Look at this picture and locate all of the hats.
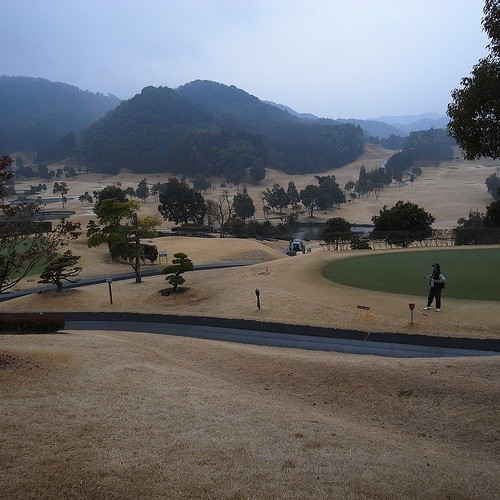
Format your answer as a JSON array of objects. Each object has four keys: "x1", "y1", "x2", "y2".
[{"x1": 432, "y1": 263, "x2": 440, "y2": 268}]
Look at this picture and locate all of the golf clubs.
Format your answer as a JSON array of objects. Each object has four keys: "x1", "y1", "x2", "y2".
[{"x1": 424, "y1": 275, "x2": 435, "y2": 281}]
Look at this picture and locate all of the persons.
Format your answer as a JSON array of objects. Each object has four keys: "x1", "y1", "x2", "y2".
[
  {"x1": 300, "y1": 242, "x2": 312, "y2": 253},
  {"x1": 288, "y1": 242, "x2": 292, "y2": 251},
  {"x1": 423, "y1": 263, "x2": 446, "y2": 312}
]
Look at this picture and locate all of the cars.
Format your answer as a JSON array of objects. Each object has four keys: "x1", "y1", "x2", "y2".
[{"x1": 293, "y1": 239, "x2": 301, "y2": 245}]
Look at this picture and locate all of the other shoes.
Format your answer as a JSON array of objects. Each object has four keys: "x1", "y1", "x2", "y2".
[
  {"x1": 423, "y1": 306, "x2": 432, "y2": 310},
  {"x1": 435, "y1": 308, "x2": 441, "y2": 312}
]
